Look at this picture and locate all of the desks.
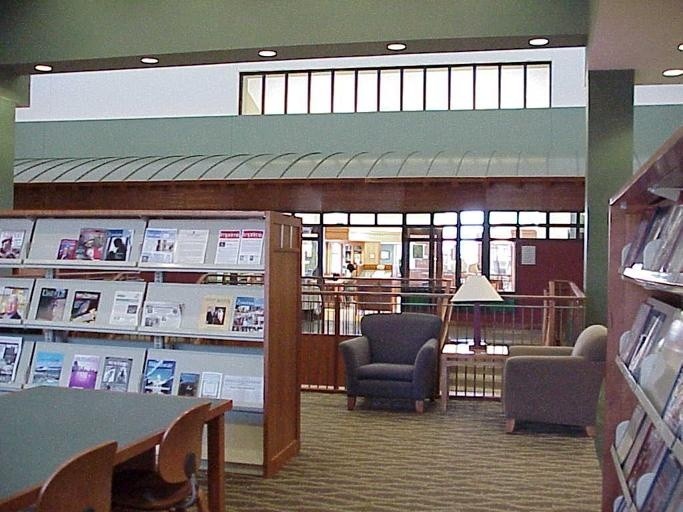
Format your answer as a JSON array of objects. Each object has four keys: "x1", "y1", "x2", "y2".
[
  {"x1": 440, "y1": 344, "x2": 510, "y2": 416},
  {"x1": 0, "y1": 385, "x2": 232, "y2": 511}
]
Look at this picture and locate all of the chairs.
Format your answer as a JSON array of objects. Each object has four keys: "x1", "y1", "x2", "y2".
[
  {"x1": 501, "y1": 324, "x2": 608, "y2": 436},
  {"x1": 111, "y1": 401, "x2": 212, "y2": 510},
  {"x1": 338, "y1": 312, "x2": 443, "y2": 414},
  {"x1": 36, "y1": 441, "x2": 118, "y2": 511}
]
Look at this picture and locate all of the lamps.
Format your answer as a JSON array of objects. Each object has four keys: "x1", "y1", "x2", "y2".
[{"x1": 449, "y1": 275, "x2": 505, "y2": 352}]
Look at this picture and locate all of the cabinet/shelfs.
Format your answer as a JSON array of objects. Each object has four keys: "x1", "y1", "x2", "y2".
[
  {"x1": 601, "y1": 125, "x2": 682, "y2": 512},
  {"x1": 1, "y1": 209, "x2": 302, "y2": 479},
  {"x1": 324, "y1": 241, "x2": 366, "y2": 277}
]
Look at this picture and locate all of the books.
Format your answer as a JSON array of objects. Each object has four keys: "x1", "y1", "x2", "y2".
[
  {"x1": 2, "y1": 226, "x2": 134, "y2": 262},
  {"x1": 615, "y1": 190, "x2": 683, "y2": 511},
  {"x1": 0, "y1": 336, "x2": 224, "y2": 398},
  {"x1": 0, "y1": 285, "x2": 264, "y2": 333}
]
